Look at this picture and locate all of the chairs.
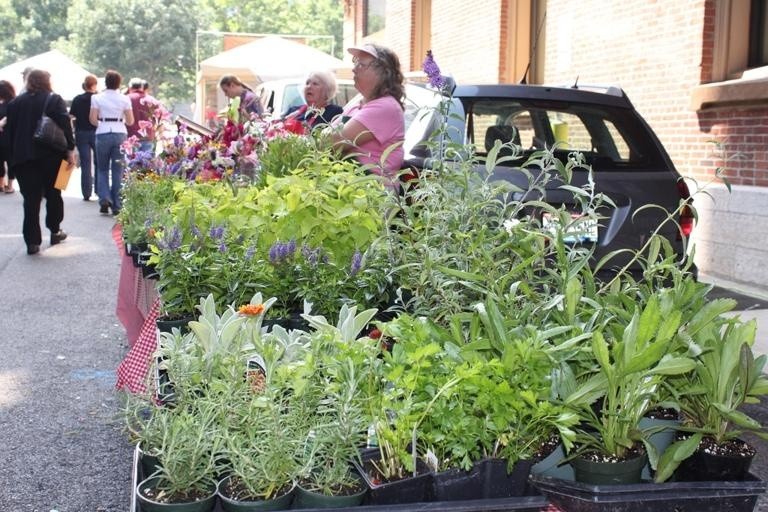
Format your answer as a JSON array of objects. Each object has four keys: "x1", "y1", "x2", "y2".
[{"x1": 484, "y1": 123, "x2": 523, "y2": 159}]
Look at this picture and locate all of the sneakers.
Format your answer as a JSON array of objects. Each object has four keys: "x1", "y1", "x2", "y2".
[
  {"x1": 26, "y1": 244, "x2": 41, "y2": 255},
  {"x1": 51, "y1": 229, "x2": 67, "y2": 245},
  {"x1": 100, "y1": 202, "x2": 110, "y2": 214}
]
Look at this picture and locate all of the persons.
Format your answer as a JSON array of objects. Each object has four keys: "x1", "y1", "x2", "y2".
[
  {"x1": 18, "y1": 68, "x2": 35, "y2": 94},
  {"x1": 139, "y1": 79, "x2": 163, "y2": 158},
  {"x1": 88, "y1": 70, "x2": 135, "y2": 214},
  {"x1": 317, "y1": 44, "x2": 404, "y2": 197},
  {"x1": 125, "y1": 78, "x2": 169, "y2": 165},
  {"x1": 68, "y1": 76, "x2": 97, "y2": 203},
  {"x1": 0, "y1": 80, "x2": 16, "y2": 194},
  {"x1": 221, "y1": 76, "x2": 263, "y2": 125},
  {"x1": 282, "y1": 70, "x2": 343, "y2": 134},
  {"x1": 4, "y1": 70, "x2": 75, "y2": 255}
]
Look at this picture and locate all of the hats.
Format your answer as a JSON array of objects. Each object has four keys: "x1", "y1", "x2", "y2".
[{"x1": 348, "y1": 43, "x2": 378, "y2": 59}]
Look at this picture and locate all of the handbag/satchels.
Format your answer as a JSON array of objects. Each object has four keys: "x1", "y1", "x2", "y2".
[{"x1": 33, "y1": 115, "x2": 68, "y2": 153}]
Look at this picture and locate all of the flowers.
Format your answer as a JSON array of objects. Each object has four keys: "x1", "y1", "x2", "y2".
[{"x1": 144, "y1": 202, "x2": 366, "y2": 313}]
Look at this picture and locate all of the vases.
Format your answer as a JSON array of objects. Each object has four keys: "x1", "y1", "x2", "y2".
[
  {"x1": 132, "y1": 183, "x2": 165, "y2": 267},
  {"x1": 124, "y1": 178, "x2": 146, "y2": 257},
  {"x1": 156, "y1": 313, "x2": 316, "y2": 335}
]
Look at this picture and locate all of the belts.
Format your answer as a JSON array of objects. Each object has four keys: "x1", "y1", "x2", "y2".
[{"x1": 99, "y1": 118, "x2": 122, "y2": 121}]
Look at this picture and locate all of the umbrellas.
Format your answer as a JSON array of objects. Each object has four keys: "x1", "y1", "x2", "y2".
[
  {"x1": 200, "y1": 32, "x2": 354, "y2": 73},
  {"x1": 1, "y1": 49, "x2": 110, "y2": 102}
]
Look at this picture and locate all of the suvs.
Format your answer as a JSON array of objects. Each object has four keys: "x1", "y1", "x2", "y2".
[{"x1": 340, "y1": 81, "x2": 703, "y2": 310}]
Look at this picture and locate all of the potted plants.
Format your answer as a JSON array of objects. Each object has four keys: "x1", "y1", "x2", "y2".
[{"x1": 141, "y1": 177, "x2": 175, "y2": 278}]
